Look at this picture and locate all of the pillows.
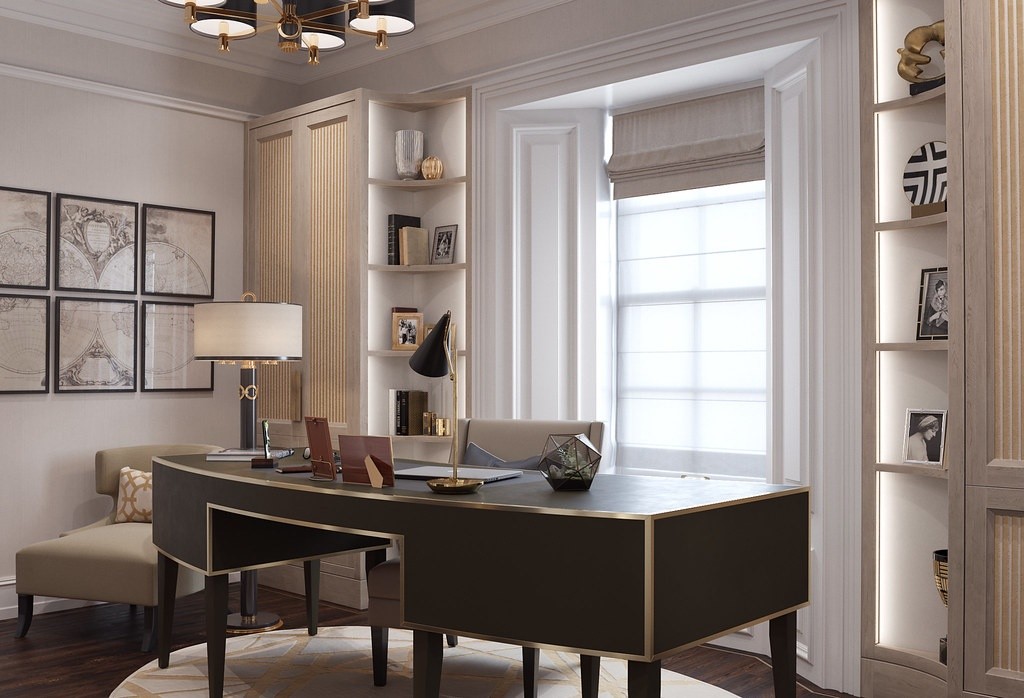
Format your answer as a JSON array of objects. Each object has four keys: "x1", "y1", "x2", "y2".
[{"x1": 114, "y1": 466, "x2": 153, "y2": 523}]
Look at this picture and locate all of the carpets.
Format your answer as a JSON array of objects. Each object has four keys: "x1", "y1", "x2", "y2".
[{"x1": 110, "y1": 626, "x2": 742, "y2": 698}]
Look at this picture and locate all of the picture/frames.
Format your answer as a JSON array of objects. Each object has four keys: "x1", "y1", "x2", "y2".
[
  {"x1": 430, "y1": 224, "x2": 458, "y2": 264},
  {"x1": 337, "y1": 435, "x2": 396, "y2": 488},
  {"x1": 0, "y1": 294, "x2": 51, "y2": 394},
  {"x1": 304, "y1": 416, "x2": 337, "y2": 480},
  {"x1": 141, "y1": 203, "x2": 216, "y2": 300},
  {"x1": 140, "y1": 301, "x2": 214, "y2": 392},
  {"x1": 916, "y1": 267, "x2": 948, "y2": 340},
  {"x1": 423, "y1": 323, "x2": 450, "y2": 350},
  {"x1": 54, "y1": 193, "x2": 139, "y2": 295},
  {"x1": 54, "y1": 296, "x2": 138, "y2": 393},
  {"x1": 392, "y1": 312, "x2": 423, "y2": 351},
  {"x1": 0, "y1": 186, "x2": 51, "y2": 290},
  {"x1": 901, "y1": 408, "x2": 948, "y2": 466}
]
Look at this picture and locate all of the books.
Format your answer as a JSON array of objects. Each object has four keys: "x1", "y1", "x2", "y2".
[
  {"x1": 206, "y1": 449, "x2": 294, "y2": 462},
  {"x1": 389, "y1": 388, "x2": 450, "y2": 435},
  {"x1": 387, "y1": 214, "x2": 430, "y2": 265},
  {"x1": 276, "y1": 465, "x2": 312, "y2": 473}
]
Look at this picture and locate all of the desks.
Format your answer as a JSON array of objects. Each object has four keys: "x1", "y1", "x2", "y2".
[{"x1": 151, "y1": 447, "x2": 811, "y2": 698}]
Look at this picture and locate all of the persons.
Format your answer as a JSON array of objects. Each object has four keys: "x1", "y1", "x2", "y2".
[
  {"x1": 399, "y1": 321, "x2": 416, "y2": 344},
  {"x1": 439, "y1": 233, "x2": 448, "y2": 256},
  {"x1": 926, "y1": 280, "x2": 948, "y2": 329},
  {"x1": 908, "y1": 415, "x2": 939, "y2": 462}
]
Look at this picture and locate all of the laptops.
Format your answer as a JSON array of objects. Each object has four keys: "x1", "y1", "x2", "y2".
[{"x1": 393, "y1": 465, "x2": 523, "y2": 482}]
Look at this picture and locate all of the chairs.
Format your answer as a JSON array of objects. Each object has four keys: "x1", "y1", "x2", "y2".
[
  {"x1": 13, "y1": 444, "x2": 227, "y2": 653},
  {"x1": 366, "y1": 418, "x2": 605, "y2": 698}
]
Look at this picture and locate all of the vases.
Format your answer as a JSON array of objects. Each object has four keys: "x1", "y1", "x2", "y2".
[{"x1": 395, "y1": 129, "x2": 424, "y2": 181}]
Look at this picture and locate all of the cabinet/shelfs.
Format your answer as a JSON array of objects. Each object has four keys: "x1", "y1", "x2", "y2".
[
  {"x1": 244, "y1": 85, "x2": 471, "y2": 454},
  {"x1": 859, "y1": 0, "x2": 1024, "y2": 698}
]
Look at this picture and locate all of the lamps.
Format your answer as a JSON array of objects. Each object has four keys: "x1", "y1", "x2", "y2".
[
  {"x1": 158, "y1": 0, "x2": 417, "y2": 66},
  {"x1": 194, "y1": 291, "x2": 303, "y2": 634},
  {"x1": 408, "y1": 309, "x2": 484, "y2": 494}
]
